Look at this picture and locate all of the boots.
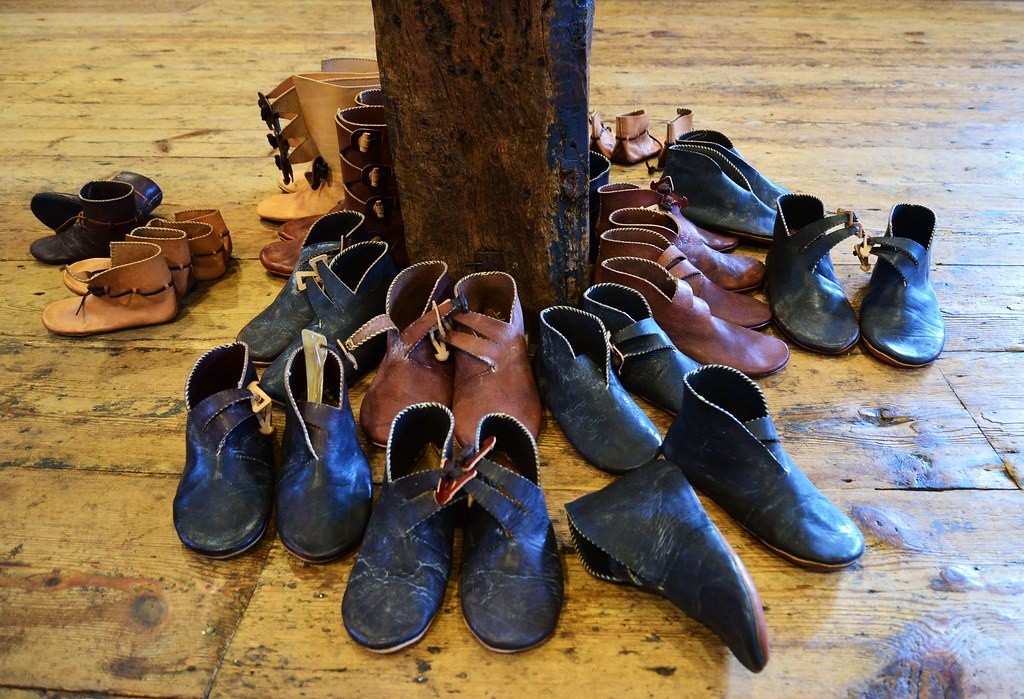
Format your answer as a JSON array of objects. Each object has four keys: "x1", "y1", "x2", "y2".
[{"x1": 31, "y1": 170, "x2": 232, "y2": 333}]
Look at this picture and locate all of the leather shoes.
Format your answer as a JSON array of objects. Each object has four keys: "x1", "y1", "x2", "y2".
[{"x1": 173, "y1": 58, "x2": 945, "y2": 672}]
